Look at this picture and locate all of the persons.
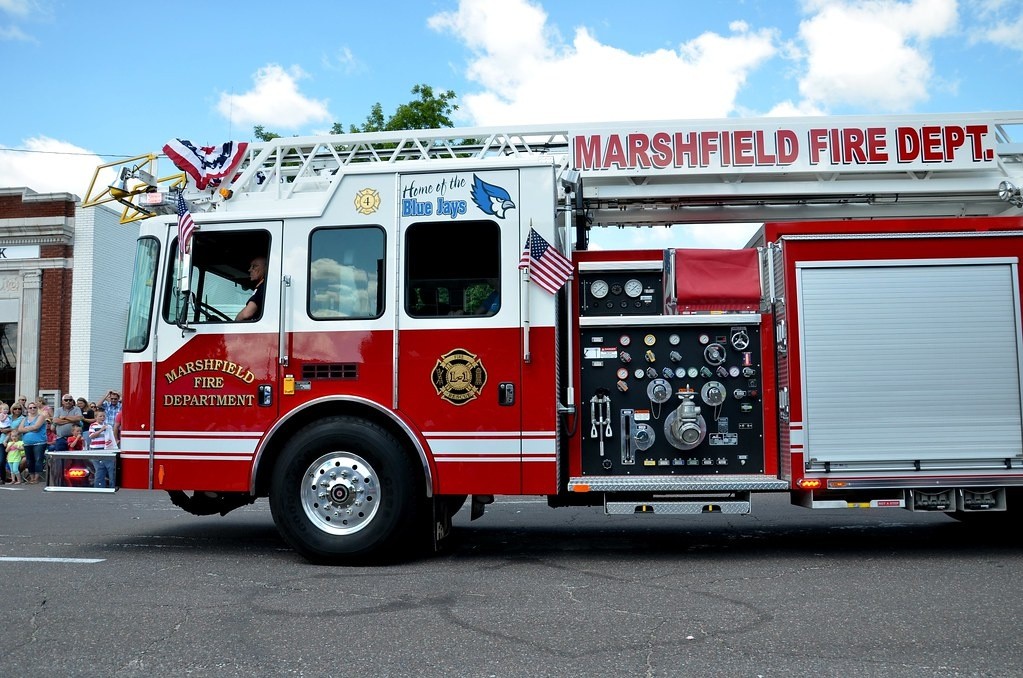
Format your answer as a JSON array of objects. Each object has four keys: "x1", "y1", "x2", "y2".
[
  {"x1": 89, "y1": 408, "x2": 120, "y2": 492},
  {"x1": 35, "y1": 397, "x2": 57, "y2": 452},
  {"x1": 68, "y1": 424, "x2": 84, "y2": 451},
  {"x1": 5, "y1": 430, "x2": 24, "y2": 484},
  {"x1": 77, "y1": 398, "x2": 96, "y2": 444},
  {"x1": 113, "y1": 412, "x2": 121, "y2": 439},
  {"x1": 0, "y1": 401, "x2": 24, "y2": 485},
  {"x1": 98, "y1": 390, "x2": 122, "y2": 426},
  {"x1": 52, "y1": 394, "x2": 83, "y2": 450},
  {"x1": 478, "y1": 279, "x2": 500, "y2": 315},
  {"x1": 17, "y1": 395, "x2": 28, "y2": 417},
  {"x1": 18, "y1": 402, "x2": 47, "y2": 484},
  {"x1": 235, "y1": 256, "x2": 266, "y2": 321}
]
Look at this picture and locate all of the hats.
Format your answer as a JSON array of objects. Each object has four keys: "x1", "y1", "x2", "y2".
[
  {"x1": 110, "y1": 390, "x2": 121, "y2": 399},
  {"x1": 45, "y1": 418, "x2": 51, "y2": 423},
  {"x1": 72, "y1": 422, "x2": 82, "y2": 427}
]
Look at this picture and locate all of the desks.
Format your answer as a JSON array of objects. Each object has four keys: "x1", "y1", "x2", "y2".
[{"x1": 44, "y1": 450, "x2": 119, "y2": 493}]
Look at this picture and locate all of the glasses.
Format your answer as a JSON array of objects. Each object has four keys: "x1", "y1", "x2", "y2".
[
  {"x1": 20, "y1": 399, "x2": 26, "y2": 401},
  {"x1": 91, "y1": 406, "x2": 96, "y2": 408},
  {"x1": 13, "y1": 408, "x2": 21, "y2": 410},
  {"x1": 111, "y1": 397, "x2": 118, "y2": 400},
  {"x1": 48, "y1": 423, "x2": 50, "y2": 424},
  {"x1": 29, "y1": 407, "x2": 36, "y2": 409},
  {"x1": 64, "y1": 399, "x2": 73, "y2": 402},
  {"x1": 249, "y1": 264, "x2": 265, "y2": 269}
]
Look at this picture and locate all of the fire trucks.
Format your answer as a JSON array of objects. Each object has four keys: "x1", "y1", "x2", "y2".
[{"x1": 82, "y1": 111, "x2": 1023, "y2": 566}]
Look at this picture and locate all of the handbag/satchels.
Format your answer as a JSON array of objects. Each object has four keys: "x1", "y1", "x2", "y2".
[{"x1": 104, "y1": 424, "x2": 119, "y2": 450}]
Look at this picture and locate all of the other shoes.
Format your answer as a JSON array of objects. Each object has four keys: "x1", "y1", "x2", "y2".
[
  {"x1": 28, "y1": 479, "x2": 39, "y2": 484},
  {"x1": 9, "y1": 480, "x2": 21, "y2": 485},
  {"x1": 3, "y1": 441, "x2": 8, "y2": 447},
  {"x1": 2, "y1": 480, "x2": 5, "y2": 484}
]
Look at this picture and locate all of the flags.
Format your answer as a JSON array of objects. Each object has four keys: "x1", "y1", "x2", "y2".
[
  {"x1": 177, "y1": 191, "x2": 195, "y2": 253},
  {"x1": 518, "y1": 227, "x2": 574, "y2": 295}
]
